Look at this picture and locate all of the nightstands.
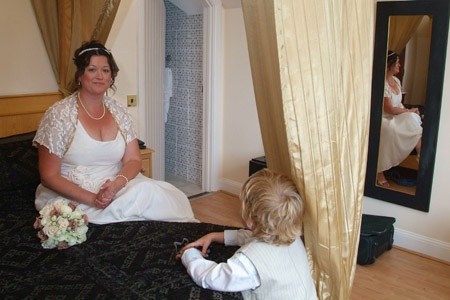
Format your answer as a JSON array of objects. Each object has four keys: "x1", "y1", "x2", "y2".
[{"x1": 135, "y1": 147, "x2": 155, "y2": 178}]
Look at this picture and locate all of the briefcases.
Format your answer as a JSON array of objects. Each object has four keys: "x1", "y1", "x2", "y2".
[{"x1": 357, "y1": 213, "x2": 396, "y2": 265}]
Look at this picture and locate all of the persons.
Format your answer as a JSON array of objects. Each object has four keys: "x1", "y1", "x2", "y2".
[
  {"x1": 376, "y1": 51, "x2": 423, "y2": 188},
  {"x1": 33, "y1": 40, "x2": 202, "y2": 224},
  {"x1": 176, "y1": 167, "x2": 319, "y2": 300}
]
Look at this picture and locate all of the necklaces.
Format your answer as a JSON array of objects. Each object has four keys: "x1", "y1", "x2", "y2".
[
  {"x1": 77, "y1": 90, "x2": 105, "y2": 120},
  {"x1": 386, "y1": 76, "x2": 395, "y2": 86}
]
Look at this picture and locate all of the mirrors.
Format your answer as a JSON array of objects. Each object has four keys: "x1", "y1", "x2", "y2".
[{"x1": 363, "y1": 0, "x2": 450, "y2": 212}]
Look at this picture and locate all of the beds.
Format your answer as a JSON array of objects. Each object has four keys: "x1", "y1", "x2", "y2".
[{"x1": 0, "y1": 92, "x2": 252, "y2": 300}]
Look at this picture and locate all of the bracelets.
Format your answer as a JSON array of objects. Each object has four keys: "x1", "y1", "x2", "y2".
[
  {"x1": 116, "y1": 175, "x2": 128, "y2": 187},
  {"x1": 407, "y1": 108, "x2": 409, "y2": 112}
]
